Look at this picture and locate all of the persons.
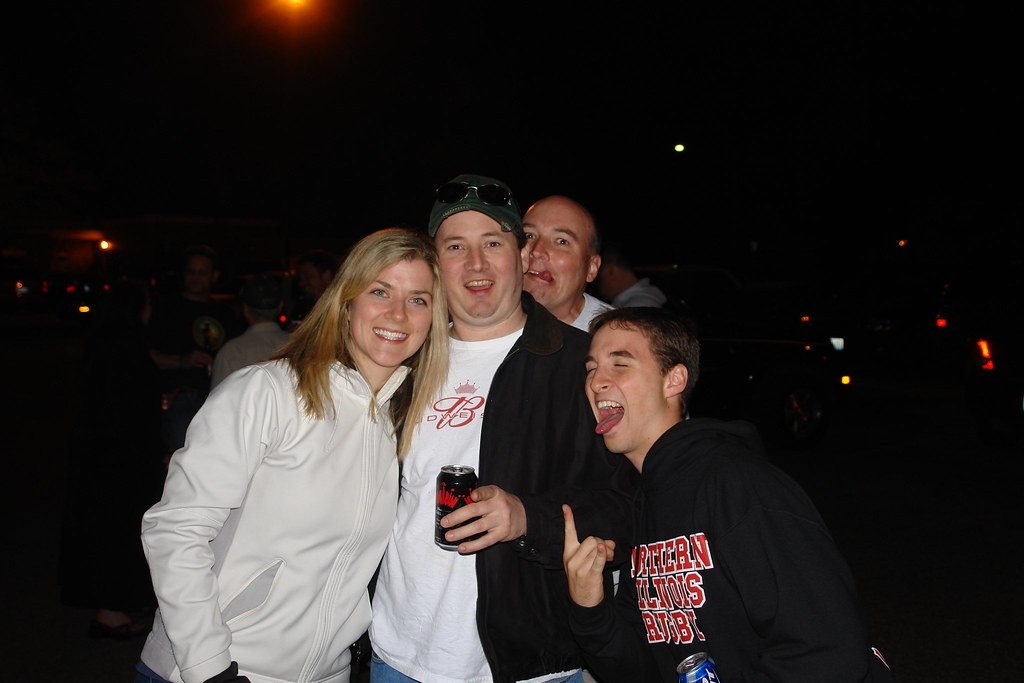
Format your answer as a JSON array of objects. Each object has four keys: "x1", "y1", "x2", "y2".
[
  {"x1": 130, "y1": 230, "x2": 449, "y2": 683},
  {"x1": 366, "y1": 177, "x2": 608, "y2": 683},
  {"x1": 563, "y1": 306, "x2": 903, "y2": 683},
  {"x1": 59, "y1": 242, "x2": 670, "y2": 638}
]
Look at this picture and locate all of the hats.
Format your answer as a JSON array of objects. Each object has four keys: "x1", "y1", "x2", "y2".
[{"x1": 429, "y1": 175, "x2": 523, "y2": 238}]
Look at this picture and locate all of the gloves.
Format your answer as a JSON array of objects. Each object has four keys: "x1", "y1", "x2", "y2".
[{"x1": 203, "y1": 662, "x2": 251, "y2": 683}]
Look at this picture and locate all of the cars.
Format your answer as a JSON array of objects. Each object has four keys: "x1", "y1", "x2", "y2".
[
  {"x1": 1, "y1": 225, "x2": 181, "y2": 331},
  {"x1": 650, "y1": 258, "x2": 861, "y2": 448}
]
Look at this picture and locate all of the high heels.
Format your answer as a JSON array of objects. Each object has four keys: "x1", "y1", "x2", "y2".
[{"x1": 88, "y1": 620, "x2": 144, "y2": 640}]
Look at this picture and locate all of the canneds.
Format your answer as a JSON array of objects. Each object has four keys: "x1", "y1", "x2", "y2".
[
  {"x1": 676, "y1": 652, "x2": 723, "y2": 683},
  {"x1": 434, "y1": 465, "x2": 479, "y2": 550}
]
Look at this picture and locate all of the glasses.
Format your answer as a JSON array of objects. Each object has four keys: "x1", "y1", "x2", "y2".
[{"x1": 436, "y1": 181, "x2": 513, "y2": 207}]
